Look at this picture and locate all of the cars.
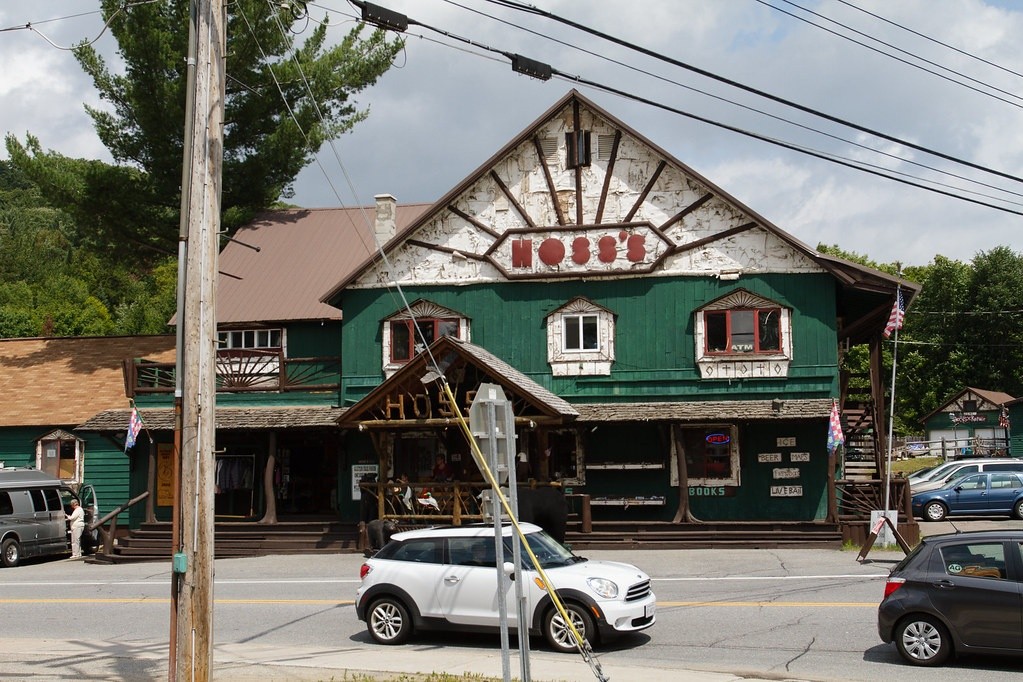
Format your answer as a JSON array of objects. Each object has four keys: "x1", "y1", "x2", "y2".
[
  {"x1": 911, "y1": 470, "x2": 1023, "y2": 519},
  {"x1": 904, "y1": 457, "x2": 1023, "y2": 486}
]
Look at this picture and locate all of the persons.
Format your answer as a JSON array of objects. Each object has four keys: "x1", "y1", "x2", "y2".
[
  {"x1": 64, "y1": 499, "x2": 84, "y2": 558},
  {"x1": 396, "y1": 472, "x2": 408, "y2": 484},
  {"x1": 433, "y1": 454, "x2": 454, "y2": 482}
]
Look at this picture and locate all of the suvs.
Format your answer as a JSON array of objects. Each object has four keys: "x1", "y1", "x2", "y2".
[
  {"x1": 356, "y1": 523, "x2": 659, "y2": 654},
  {"x1": 878, "y1": 532, "x2": 1023, "y2": 668}
]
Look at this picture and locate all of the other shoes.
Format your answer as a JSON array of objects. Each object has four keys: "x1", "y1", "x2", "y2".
[{"x1": 70, "y1": 555, "x2": 82, "y2": 559}]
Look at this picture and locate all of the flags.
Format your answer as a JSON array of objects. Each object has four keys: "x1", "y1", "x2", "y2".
[
  {"x1": 124, "y1": 407, "x2": 142, "y2": 453},
  {"x1": 827, "y1": 402, "x2": 844, "y2": 455},
  {"x1": 882, "y1": 290, "x2": 904, "y2": 339}
]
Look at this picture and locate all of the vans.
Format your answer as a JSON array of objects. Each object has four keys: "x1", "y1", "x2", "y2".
[
  {"x1": 908, "y1": 461, "x2": 1023, "y2": 496},
  {"x1": 0, "y1": 470, "x2": 99, "y2": 567}
]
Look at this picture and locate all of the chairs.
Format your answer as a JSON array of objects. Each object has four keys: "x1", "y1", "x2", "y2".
[{"x1": 453, "y1": 549, "x2": 473, "y2": 564}]
